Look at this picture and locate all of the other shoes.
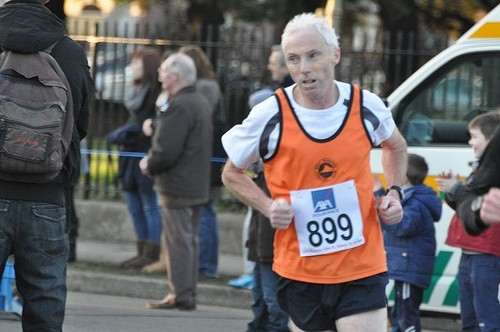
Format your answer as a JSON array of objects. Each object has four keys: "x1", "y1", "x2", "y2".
[
  {"x1": 147, "y1": 297, "x2": 191, "y2": 310},
  {"x1": 144, "y1": 259, "x2": 167, "y2": 273},
  {"x1": 198, "y1": 273, "x2": 215, "y2": 283}
]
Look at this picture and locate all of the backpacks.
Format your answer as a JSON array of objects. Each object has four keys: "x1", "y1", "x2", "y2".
[{"x1": 0, "y1": 34, "x2": 74, "y2": 184}]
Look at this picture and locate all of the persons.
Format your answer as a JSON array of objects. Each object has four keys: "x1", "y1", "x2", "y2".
[
  {"x1": 372, "y1": 153, "x2": 442, "y2": 332},
  {"x1": 106, "y1": 12, "x2": 409, "y2": 332},
  {"x1": 456, "y1": 126, "x2": 500, "y2": 236},
  {"x1": 0, "y1": 0, "x2": 97, "y2": 332},
  {"x1": 444, "y1": 110, "x2": 500, "y2": 332}
]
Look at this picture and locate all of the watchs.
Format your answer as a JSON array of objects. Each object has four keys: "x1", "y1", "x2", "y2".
[
  {"x1": 470, "y1": 195, "x2": 491, "y2": 231},
  {"x1": 384, "y1": 186, "x2": 405, "y2": 201}
]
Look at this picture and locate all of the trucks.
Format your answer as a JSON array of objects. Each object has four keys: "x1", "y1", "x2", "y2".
[{"x1": 369, "y1": 5, "x2": 500, "y2": 315}]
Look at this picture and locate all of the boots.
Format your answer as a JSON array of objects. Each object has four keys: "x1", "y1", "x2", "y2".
[{"x1": 121, "y1": 240, "x2": 160, "y2": 269}]
